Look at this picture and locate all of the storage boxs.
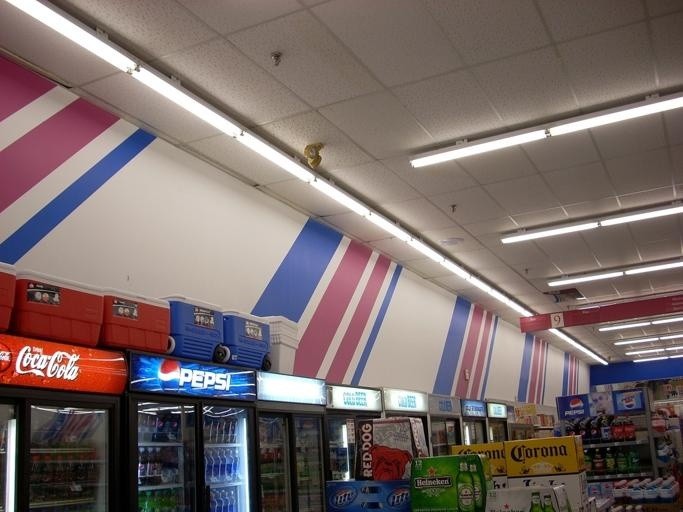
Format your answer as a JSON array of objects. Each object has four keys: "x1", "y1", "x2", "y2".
[{"x1": 323, "y1": 429, "x2": 595, "y2": 512}]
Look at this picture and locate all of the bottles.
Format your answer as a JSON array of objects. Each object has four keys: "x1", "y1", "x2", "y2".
[
  {"x1": 259, "y1": 441, "x2": 310, "y2": 512},
  {"x1": 29, "y1": 451, "x2": 97, "y2": 504},
  {"x1": 555, "y1": 414, "x2": 640, "y2": 476},
  {"x1": 454, "y1": 455, "x2": 484, "y2": 511},
  {"x1": 132, "y1": 407, "x2": 237, "y2": 511}
]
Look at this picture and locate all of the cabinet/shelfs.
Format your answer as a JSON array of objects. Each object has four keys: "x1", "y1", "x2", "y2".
[{"x1": 506, "y1": 388, "x2": 683, "y2": 510}]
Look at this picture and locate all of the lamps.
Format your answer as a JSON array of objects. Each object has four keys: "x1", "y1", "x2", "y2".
[
  {"x1": 132, "y1": 67, "x2": 241, "y2": 138},
  {"x1": 239, "y1": 133, "x2": 315, "y2": 182},
  {"x1": 408, "y1": 126, "x2": 547, "y2": 168},
  {"x1": 623, "y1": 260, "x2": 683, "y2": 276},
  {"x1": 310, "y1": 179, "x2": 369, "y2": 217},
  {"x1": 2, "y1": 0, "x2": 137, "y2": 74},
  {"x1": 546, "y1": 271, "x2": 624, "y2": 288},
  {"x1": 547, "y1": 317, "x2": 681, "y2": 363},
  {"x1": 549, "y1": 91, "x2": 683, "y2": 137},
  {"x1": 406, "y1": 238, "x2": 534, "y2": 321},
  {"x1": 368, "y1": 213, "x2": 412, "y2": 244},
  {"x1": 499, "y1": 219, "x2": 599, "y2": 245},
  {"x1": 599, "y1": 205, "x2": 683, "y2": 228}
]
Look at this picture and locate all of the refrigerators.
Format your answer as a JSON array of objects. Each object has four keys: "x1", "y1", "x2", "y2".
[
  {"x1": 0, "y1": 334, "x2": 128, "y2": 512},
  {"x1": 331, "y1": 381, "x2": 385, "y2": 510},
  {"x1": 462, "y1": 400, "x2": 488, "y2": 448},
  {"x1": 387, "y1": 386, "x2": 429, "y2": 450},
  {"x1": 129, "y1": 361, "x2": 256, "y2": 512},
  {"x1": 257, "y1": 377, "x2": 330, "y2": 508},
  {"x1": 427, "y1": 395, "x2": 461, "y2": 455},
  {"x1": 489, "y1": 401, "x2": 515, "y2": 441}
]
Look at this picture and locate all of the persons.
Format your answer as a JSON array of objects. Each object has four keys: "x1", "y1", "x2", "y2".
[{"x1": 590, "y1": 392, "x2": 612, "y2": 415}]
